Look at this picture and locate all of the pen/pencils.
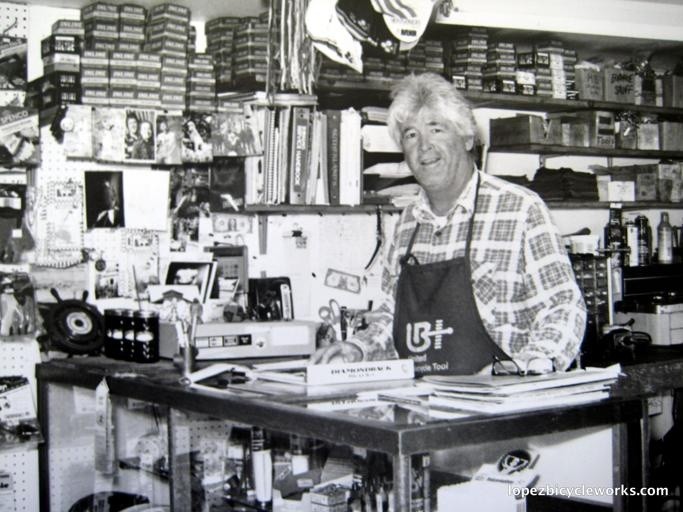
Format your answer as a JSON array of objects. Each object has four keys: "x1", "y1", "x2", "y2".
[{"x1": 339, "y1": 307, "x2": 349, "y2": 347}]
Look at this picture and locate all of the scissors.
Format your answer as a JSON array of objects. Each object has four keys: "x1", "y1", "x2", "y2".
[{"x1": 320, "y1": 300, "x2": 342, "y2": 342}]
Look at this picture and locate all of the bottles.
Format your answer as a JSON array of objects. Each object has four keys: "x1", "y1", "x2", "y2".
[
  {"x1": 344, "y1": 480, "x2": 388, "y2": 511},
  {"x1": 603, "y1": 204, "x2": 675, "y2": 268}
]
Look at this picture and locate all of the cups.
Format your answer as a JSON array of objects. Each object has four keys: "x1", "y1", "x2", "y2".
[{"x1": 102, "y1": 308, "x2": 160, "y2": 364}]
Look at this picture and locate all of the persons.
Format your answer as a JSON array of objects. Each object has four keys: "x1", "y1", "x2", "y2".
[
  {"x1": 126, "y1": 112, "x2": 154, "y2": 159},
  {"x1": 95, "y1": 176, "x2": 115, "y2": 225},
  {"x1": 157, "y1": 119, "x2": 175, "y2": 165},
  {"x1": 307, "y1": 71, "x2": 587, "y2": 376},
  {"x1": 0, "y1": 276, "x2": 34, "y2": 336}
]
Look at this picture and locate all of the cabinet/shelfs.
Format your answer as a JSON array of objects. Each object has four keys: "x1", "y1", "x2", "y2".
[
  {"x1": 36, "y1": 362, "x2": 644, "y2": 512},
  {"x1": 241, "y1": 22, "x2": 683, "y2": 214}
]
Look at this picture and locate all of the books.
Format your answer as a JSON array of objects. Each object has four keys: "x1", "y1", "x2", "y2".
[
  {"x1": 212, "y1": 90, "x2": 419, "y2": 207},
  {"x1": 380, "y1": 363, "x2": 620, "y2": 422}
]
